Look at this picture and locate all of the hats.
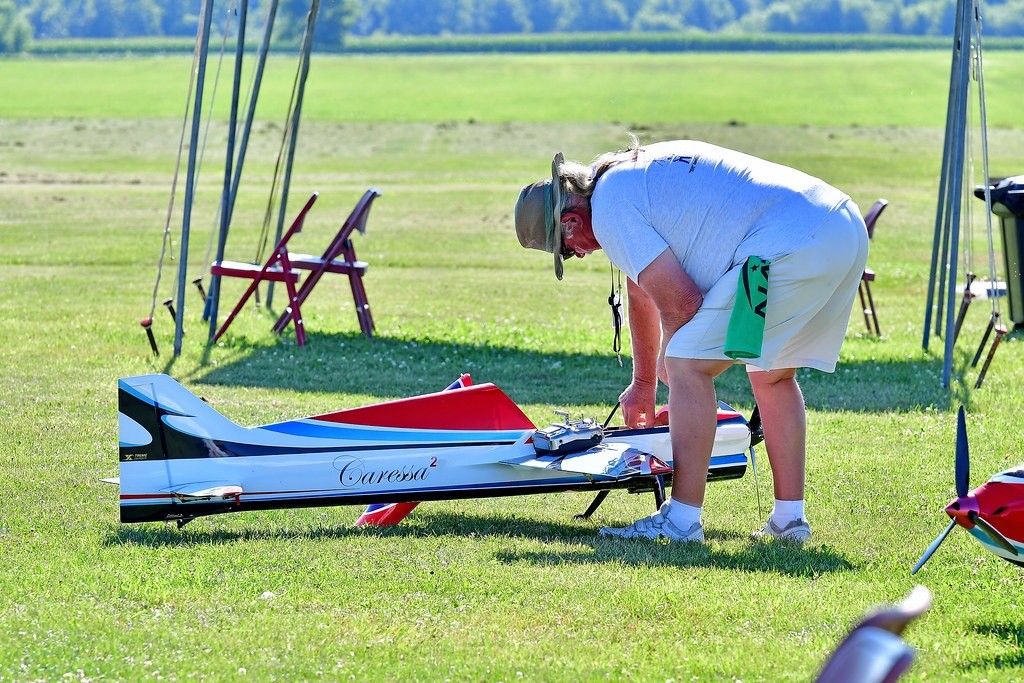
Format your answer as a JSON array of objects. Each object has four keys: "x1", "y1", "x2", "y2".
[{"x1": 515, "y1": 152, "x2": 564, "y2": 281}]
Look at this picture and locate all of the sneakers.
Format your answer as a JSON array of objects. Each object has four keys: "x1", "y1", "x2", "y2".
[
  {"x1": 753, "y1": 513, "x2": 811, "y2": 542},
  {"x1": 599, "y1": 500, "x2": 703, "y2": 542}
]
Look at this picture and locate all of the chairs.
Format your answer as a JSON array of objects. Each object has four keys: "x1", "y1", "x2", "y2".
[
  {"x1": 210, "y1": 192, "x2": 319, "y2": 347},
  {"x1": 857, "y1": 199, "x2": 889, "y2": 336},
  {"x1": 274, "y1": 187, "x2": 383, "y2": 337}
]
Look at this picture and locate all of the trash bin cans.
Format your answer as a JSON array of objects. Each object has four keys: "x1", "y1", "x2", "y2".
[{"x1": 973, "y1": 173, "x2": 1024, "y2": 325}]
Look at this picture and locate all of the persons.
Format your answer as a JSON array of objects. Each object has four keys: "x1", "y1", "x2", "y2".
[{"x1": 515, "y1": 140, "x2": 868, "y2": 543}]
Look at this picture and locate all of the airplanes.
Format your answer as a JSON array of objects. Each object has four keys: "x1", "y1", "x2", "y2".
[
  {"x1": 103, "y1": 373, "x2": 754, "y2": 526},
  {"x1": 161, "y1": 485, "x2": 243, "y2": 502},
  {"x1": 909, "y1": 404, "x2": 1023, "y2": 575}
]
